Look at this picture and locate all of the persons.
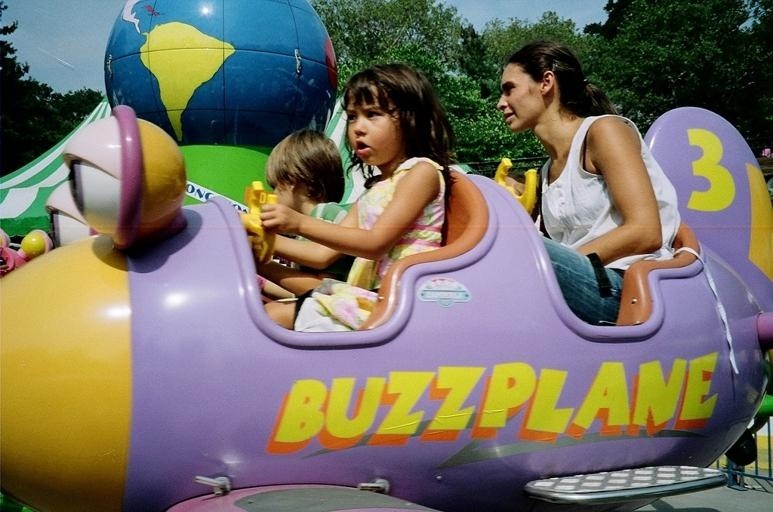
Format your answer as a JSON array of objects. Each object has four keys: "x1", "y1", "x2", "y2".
[
  {"x1": 265, "y1": 129, "x2": 351, "y2": 297},
  {"x1": 496, "y1": 41, "x2": 679, "y2": 324},
  {"x1": 262, "y1": 62, "x2": 456, "y2": 336}
]
